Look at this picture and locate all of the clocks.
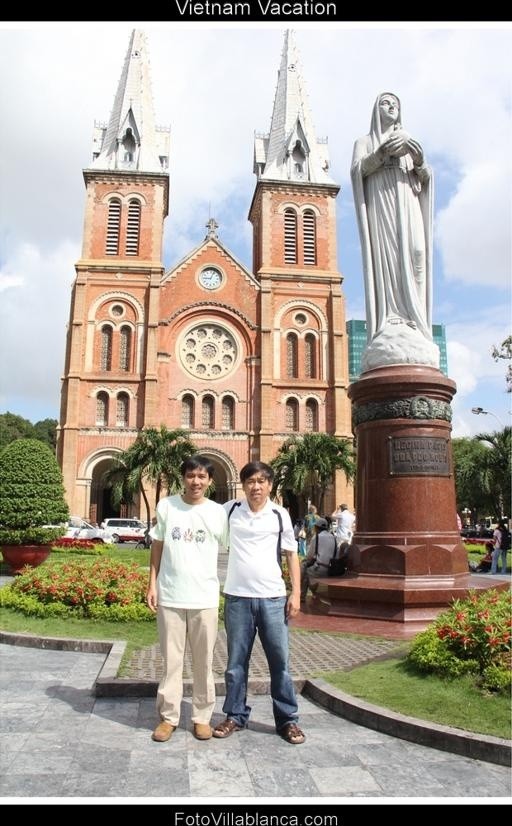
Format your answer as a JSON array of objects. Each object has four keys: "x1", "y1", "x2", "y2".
[{"x1": 198, "y1": 263, "x2": 224, "y2": 290}]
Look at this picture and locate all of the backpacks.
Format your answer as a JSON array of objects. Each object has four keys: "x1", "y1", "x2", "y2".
[{"x1": 496, "y1": 527, "x2": 512, "y2": 550}]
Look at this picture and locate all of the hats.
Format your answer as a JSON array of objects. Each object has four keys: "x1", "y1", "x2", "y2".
[
  {"x1": 313, "y1": 517, "x2": 328, "y2": 529},
  {"x1": 339, "y1": 503, "x2": 348, "y2": 510}
]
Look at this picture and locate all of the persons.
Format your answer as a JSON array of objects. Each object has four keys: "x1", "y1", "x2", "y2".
[
  {"x1": 294, "y1": 503, "x2": 356, "y2": 604},
  {"x1": 151, "y1": 460, "x2": 305, "y2": 745},
  {"x1": 488, "y1": 520, "x2": 508, "y2": 575},
  {"x1": 145, "y1": 455, "x2": 230, "y2": 742},
  {"x1": 480, "y1": 525, "x2": 487, "y2": 537},
  {"x1": 476, "y1": 542, "x2": 495, "y2": 573},
  {"x1": 348, "y1": 91, "x2": 440, "y2": 371}
]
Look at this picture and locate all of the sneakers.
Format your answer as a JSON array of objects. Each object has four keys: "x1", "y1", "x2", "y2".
[
  {"x1": 192, "y1": 722, "x2": 211, "y2": 740},
  {"x1": 152, "y1": 720, "x2": 176, "y2": 742}
]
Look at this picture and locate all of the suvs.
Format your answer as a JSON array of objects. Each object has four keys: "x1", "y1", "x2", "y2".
[{"x1": 101, "y1": 516, "x2": 148, "y2": 544}]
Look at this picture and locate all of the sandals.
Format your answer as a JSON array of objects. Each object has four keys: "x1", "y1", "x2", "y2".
[
  {"x1": 212, "y1": 718, "x2": 249, "y2": 738},
  {"x1": 276, "y1": 721, "x2": 306, "y2": 744}
]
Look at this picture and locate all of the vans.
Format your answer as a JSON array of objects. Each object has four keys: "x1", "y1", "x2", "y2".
[
  {"x1": 482, "y1": 516, "x2": 509, "y2": 533},
  {"x1": 40, "y1": 511, "x2": 116, "y2": 551}
]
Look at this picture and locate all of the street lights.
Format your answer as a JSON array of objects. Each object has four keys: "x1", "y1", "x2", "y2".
[
  {"x1": 471, "y1": 406, "x2": 506, "y2": 438},
  {"x1": 461, "y1": 507, "x2": 474, "y2": 537}
]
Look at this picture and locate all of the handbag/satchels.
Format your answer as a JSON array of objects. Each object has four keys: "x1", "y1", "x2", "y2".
[
  {"x1": 326, "y1": 557, "x2": 345, "y2": 578},
  {"x1": 307, "y1": 558, "x2": 316, "y2": 567}
]
